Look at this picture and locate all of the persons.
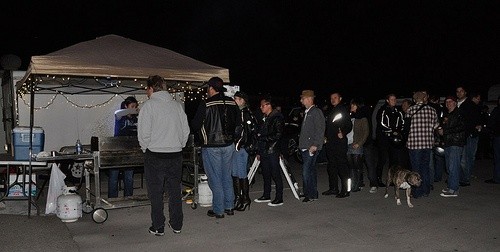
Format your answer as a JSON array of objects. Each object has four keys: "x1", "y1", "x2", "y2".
[
  {"x1": 345, "y1": 99, "x2": 369, "y2": 192},
  {"x1": 322, "y1": 92, "x2": 353, "y2": 198},
  {"x1": 137, "y1": 75, "x2": 190, "y2": 235},
  {"x1": 231, "y1": 90, "x2": 257, "y2": 211},
  {"x1": 298, "y1": 90, "x2": 325, "y2": 203},
  {"x1": 254, "y1": 95, "x2": 285, "y2": 207},
  {"x1": 193, "y1": 76, "x2": 241, "y2": 218},
  {"x1": 370, "y1": 86, "x2": 500, "y2": 199},
  {"x1": 107, "y1": 97, "x2": 140, "y2": 198}
]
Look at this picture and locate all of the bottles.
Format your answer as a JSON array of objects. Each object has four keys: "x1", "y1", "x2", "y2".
[{"x1": 76, "y1": 140, "x2": 82, "y2": 155}]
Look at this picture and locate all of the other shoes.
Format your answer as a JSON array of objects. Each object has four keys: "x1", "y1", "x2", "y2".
[
  {"x1": 322, "y1": 190, "x2": 339, "y2": 195},
  {"x1": 303, "y1": 197, "x2": 314, "y2": 203},
  {"x1": 377, "y1": 177, "x2": 386, "y2": 187},
  {"x1": 369, "y1": 186, "x2": 378, "y2": 193},
  {"x1": 336, "y1": 191, "x2": 350, "y2": 198},
  {"x1": 360, "y1": 184, "x2": 365, "y2": 187},
  {"x1": 459, "y1": 182, "x2": 470, "y2": 186},
  {"x1": 300, "y1": 195, "x2": 318, "y2": 200},
  {"x1": 440, "y1": 188, "x2": 458, "y2": 197},
  {"x1": 370, "y1": 178, "x2": 378, "y2": 188},
  {"x1": 433, "y1": 178, "x2": 441, "y2": 182},
  {"x1": 484, "y1": 179, "x2": 500, "y2": 184}
]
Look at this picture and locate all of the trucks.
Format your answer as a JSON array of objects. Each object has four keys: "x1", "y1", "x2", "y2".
[{"x1": 12, "y1": 71, "x2": 241, "y2": 179}]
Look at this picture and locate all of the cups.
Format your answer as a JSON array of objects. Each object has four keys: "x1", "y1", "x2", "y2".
[{"x1": 309, "y1": 149, "x2": 314, "y2": 156}]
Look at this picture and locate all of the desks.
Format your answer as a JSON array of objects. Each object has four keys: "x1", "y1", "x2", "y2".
[{"x1": 0, "y1": 155, "x2": 92, "y2": 218}]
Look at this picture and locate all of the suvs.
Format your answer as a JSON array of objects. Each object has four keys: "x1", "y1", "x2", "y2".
[{"x1": 287, "y1": 107, "x2": 330, "y2": 164}]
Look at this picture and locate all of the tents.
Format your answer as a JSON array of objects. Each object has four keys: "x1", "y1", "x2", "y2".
[{"x1": 16, "y1": 33, "x2": 231, "y2": 215}]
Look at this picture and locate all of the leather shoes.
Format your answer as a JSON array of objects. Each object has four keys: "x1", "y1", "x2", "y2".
[
  {"x1": 207, "y1": 210, "x2": 224, "y2": 218},
  {"x1": 225, "y1": 208, "x2": 234, "y2": 215}
]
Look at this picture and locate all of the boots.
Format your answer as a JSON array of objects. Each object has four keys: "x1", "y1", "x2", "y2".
[
  {"x1": 351, "y1": 169, "x2": 356, "y2": 190},
  {"x1": 237, "y1": 177, "x2": 251, "y2": 211},
  {"x1": 352, "y1": 169, "x2": 363, "y2": 191},
  {"x1": 232, "y1": 176, "x2": 242, "y2": 210}
]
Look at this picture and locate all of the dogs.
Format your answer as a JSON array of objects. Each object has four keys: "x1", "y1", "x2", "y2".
[{"x1": 383, "y1": 165, "x2": 423, "y2": 208}]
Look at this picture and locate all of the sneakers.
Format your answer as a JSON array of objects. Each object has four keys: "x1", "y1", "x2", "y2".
[
  {"x1": 168, "y1": 220, "x2": 181, "y2": 233},
  {"x1": 149, "y1": 227, "x2": 164, "y2": 236},
  {"x1": 267, "y1": 198, "x2": 284, "y2": 207},
  {"x1": 254, "y1": 195, "x2": 271, "y2": 203}
]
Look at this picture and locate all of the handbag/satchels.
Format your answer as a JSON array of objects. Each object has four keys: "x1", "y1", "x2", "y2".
[{"x1": 398, "y1": 103, "x2": 427, "y2": 135}]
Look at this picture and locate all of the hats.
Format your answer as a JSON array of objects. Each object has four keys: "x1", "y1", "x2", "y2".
[
  {"x1": 300, "y1": 90, "x2": 315, "y2": 98},
  {"x1": 443, "y1": 96, "x2": 457, "y2": 103},
  {"x1": 234, "y1": 91, "x2": 248, "y2": 101},
  {"x1": 208, "y1": 77, "x2": 227, "y2": 92},
  {"x1": 413, "y1": 92, "x2": 425, "y2": 101}
]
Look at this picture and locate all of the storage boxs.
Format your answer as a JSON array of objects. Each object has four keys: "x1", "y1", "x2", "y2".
[{"x1": 11, "y1": 127, "x2": 44, "y2": 160}]
